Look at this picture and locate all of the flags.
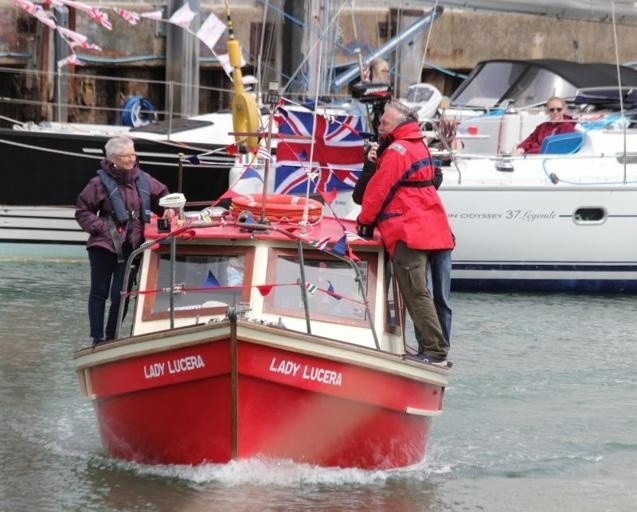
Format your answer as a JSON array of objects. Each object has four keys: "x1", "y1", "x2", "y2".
[
  {"x1": 16, "y1": 0, "x2": 257, "y2": 89},
  {"x1": 273, "y1": 100, "x2": 369, "y2": 208}
]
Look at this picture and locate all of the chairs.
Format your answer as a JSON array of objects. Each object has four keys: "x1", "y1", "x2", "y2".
[{"x1": 539, "y1": 133, "x2": 584, "y2": 155}]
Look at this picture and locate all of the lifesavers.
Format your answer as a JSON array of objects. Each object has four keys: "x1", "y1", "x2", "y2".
[
  {"x1": 229, "y1": 195, "x2": 323, "y2": 223},
  {"x1": 121, "y1": 96, "x2": 156, "y2": 126},
  {"x1": 233, "y1": 94, "x2": 258, "y2": 150}
]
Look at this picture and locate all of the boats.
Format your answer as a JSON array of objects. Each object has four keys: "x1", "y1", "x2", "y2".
[
  {"x1": 72, "y1": 214, "x2": 449, "y2": 470},
  {"x1": 0, "y1": 1, "x2": 637, "y2": 295}
]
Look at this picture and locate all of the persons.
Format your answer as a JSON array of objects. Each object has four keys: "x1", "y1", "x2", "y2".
[
  {"x1": 119, "y1": 74, "x2": 150, "y2": 103},
  {"x1": 514, "y1": 95, "x2": 576, "y2": 160},
  {"x1": 350, "y1": 58, "x2": 393, "y2": 134},
  {"x1": 73, "y1": 130, "x2": 180, "y2": 346},
  {"x1": 346, "y1": 105, "x2": 456, "y2": 348},
  {"x1": 355, "y1": 99, "x2": 461, "y2": 365}
]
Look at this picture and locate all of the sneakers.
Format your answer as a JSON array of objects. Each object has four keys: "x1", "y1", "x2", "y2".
[
  {"x1": 92, "y1": 339, "x2": 105, "y2": 345},
  {"x1": 402, "y1": 351, "x2": 448, "y2": 368}
]
